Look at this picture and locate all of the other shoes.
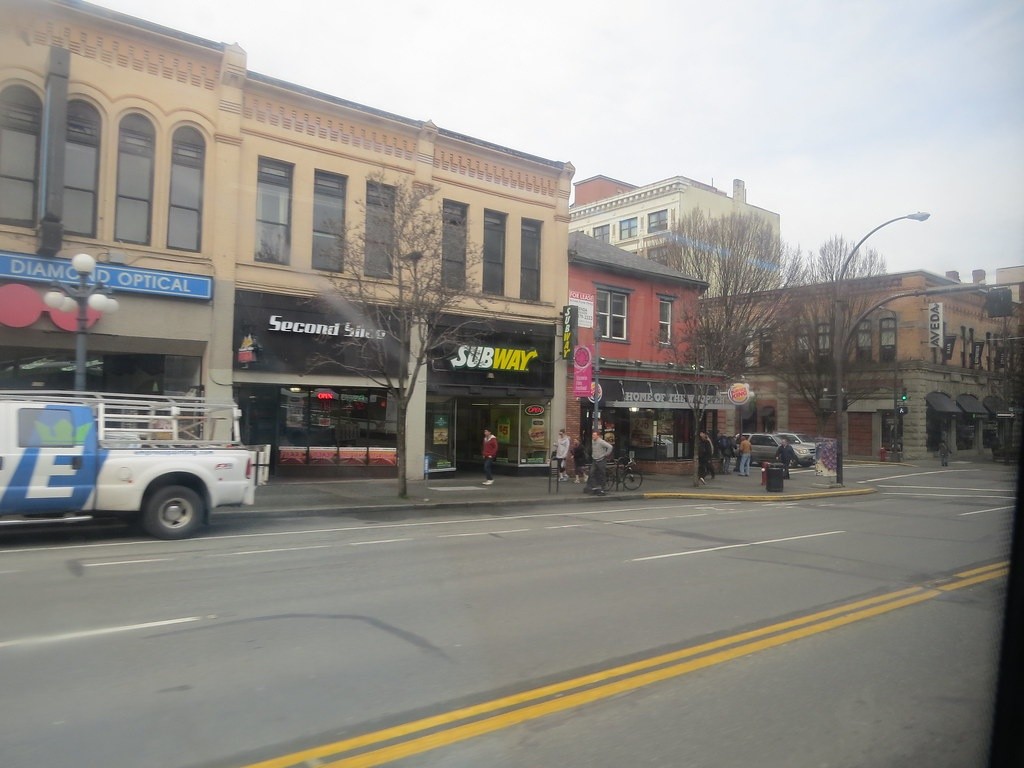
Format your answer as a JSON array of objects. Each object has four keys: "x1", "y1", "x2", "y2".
[
  {"x1": 482, "y1": 479, "x2": 494, "y2": 485},
  {"x1": 558, "y1": 474, "x2": 568, "y2": 482},
  {"x1": 574, "y1": 479, "x2": 580, "y2": 483},
  {"x1": 700, "y1": 477, "x2": 706, "y2": 484},
  {"x1": 584, "y1": 475, "x2": 589, "y2": 481}
]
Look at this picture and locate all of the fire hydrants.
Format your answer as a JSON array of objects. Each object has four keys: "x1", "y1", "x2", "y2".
[{"x1": 760, "y1": 462, "x2": 769, "y2": 485}]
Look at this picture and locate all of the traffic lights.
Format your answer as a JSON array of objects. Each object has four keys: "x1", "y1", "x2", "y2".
[
  {"x1": 901, "y1": 387, "x2": 907, "y2": 401},
  {"x1": 897, "y1": 407, "x2": 907, "y2": 416}
]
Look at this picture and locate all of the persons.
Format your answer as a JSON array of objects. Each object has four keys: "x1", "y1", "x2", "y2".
[
  {"x1": 723, "y1": 436, "x2": 737, "y2": 475},
  {"x1": 571, "y1": 438, "x2": 589, "y2": 483},
  {"x1": 588, "y1": 428, "x2": 613, "y2": 495},
  {"x1": 776, "y1": 439, "x2": 798, "y2": 479},
  {"x1": 482, "y1": 429, "x2": 498, "y2": 485},
  {"x1": 939, "y1": 441, "x2": 952, "y2": 466},
  {"x1": 739, "y1": 436, "x2": 752, "y2": 476},
  {"x1": 554, "y1": 429, "x2": 570, "y2": 480},
  {"x1": 699, "y1": 431, "x2": 714, "y2": 484}
]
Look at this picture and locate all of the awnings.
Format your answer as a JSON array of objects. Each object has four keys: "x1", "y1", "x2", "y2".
[
  {"x1": 983, "y1": 397, "x2": 1014, "y2": 415},
  {"x1": 596, "y1": 379, "x2": 721, "y2": 409},
  {"x1": 928, "y1": 393, "x2": 963, "y2": 414},
  {"x1": 957, "y1": 395, "x2": 990, "y2": 414}
]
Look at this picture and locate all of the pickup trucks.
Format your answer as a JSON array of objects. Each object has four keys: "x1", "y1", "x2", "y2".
[{"x1": 0, "y1": 400, "x2": 271, "y2": 537}]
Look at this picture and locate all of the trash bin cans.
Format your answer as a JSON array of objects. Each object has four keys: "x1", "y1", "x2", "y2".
[
  {"x1": 766, "y1": 463, "x2": 784, "y2": 491},
  {"x1": 653, "y1": 441, "x2": 667, "y2": 461}
]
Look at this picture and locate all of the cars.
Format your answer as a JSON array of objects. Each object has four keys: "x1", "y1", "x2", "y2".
[
  {"x1": 778, "y1": 433, "x2": 818, "y2": 463},
  {"x1": 733, "y1": 433, "x2": 813, "y2": 468}
]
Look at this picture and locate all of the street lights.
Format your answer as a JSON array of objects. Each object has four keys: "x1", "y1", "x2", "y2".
[
  {"x1": 819, "y1": 212, "x2": 930, "y2": 487},
  {"x1": 47, "y1": 253, "x2": 120, "y2": 393}
]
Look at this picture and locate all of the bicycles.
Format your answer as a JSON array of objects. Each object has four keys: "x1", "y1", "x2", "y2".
[{"x1": 605, "y1": 458, "x2": 644, "y2": 491}]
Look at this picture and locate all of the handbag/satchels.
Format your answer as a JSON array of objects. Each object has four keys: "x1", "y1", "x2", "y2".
[{"x1": 552, "y1": 450, "x2": 557, "y2": 460}]
